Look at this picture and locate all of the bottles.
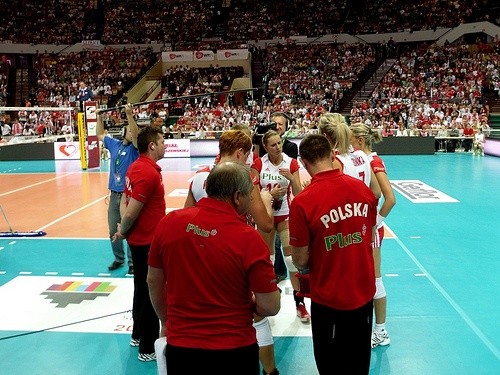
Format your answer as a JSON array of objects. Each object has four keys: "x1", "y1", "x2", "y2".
[{"x1": 271, "y1": 176, "x2": 289, "y2": 210}]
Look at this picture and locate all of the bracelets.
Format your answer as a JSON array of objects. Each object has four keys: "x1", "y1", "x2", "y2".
[{"x1": 375, "y1": 214, "x2": 385, "y2": 226}]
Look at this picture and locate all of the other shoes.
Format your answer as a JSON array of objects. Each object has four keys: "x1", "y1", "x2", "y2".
[
  {"x1": 108, "y1": 262, "x2": 120, "y2": 270},
  {"x1": 128, "y1": 266, "x2": 133, "y2": 274}
]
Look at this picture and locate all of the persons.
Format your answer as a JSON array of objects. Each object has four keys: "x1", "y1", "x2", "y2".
[
  {"x1": 147, "y1": 162, "x2": 280, "y2": 375},
  {"x1": 0, "y1": 0, "x2": 500, "y2": 375}
]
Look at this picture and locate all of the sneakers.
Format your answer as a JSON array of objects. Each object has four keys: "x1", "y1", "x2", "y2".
[
  {"x1": 296, "y1": 302, "x2": 310, "y2": 321},
  {"x1": 137, "y1": 353, "x2": 156, "y2": 361},
  {"x1": 371, "y1": 331, "x2": 390, "y2": 348},
  {"x1": 130, "y1": 338, "x2": 140, "y2": 346}
]
